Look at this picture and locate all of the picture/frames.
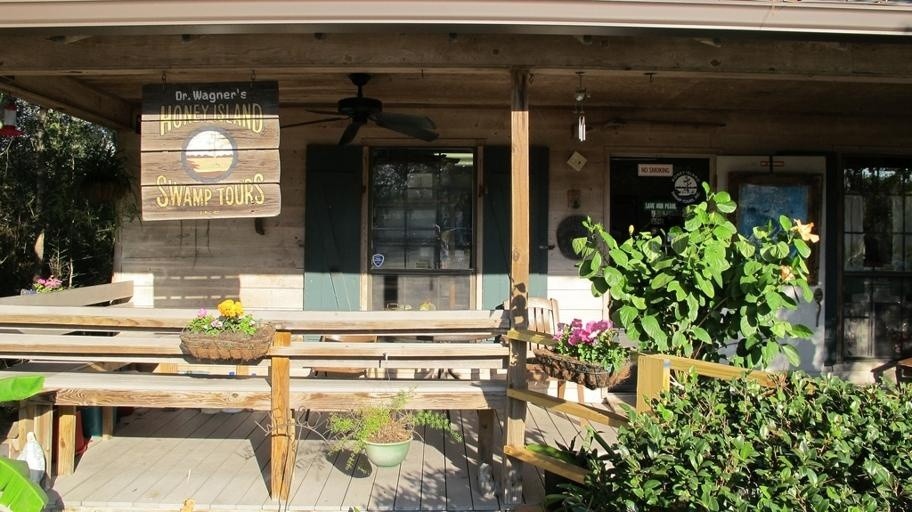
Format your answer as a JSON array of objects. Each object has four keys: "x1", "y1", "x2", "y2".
[{"x1": 723, "y1": 169, "x2": 823, "y2": 288}]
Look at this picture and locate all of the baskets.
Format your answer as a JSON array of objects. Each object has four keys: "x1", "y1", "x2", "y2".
[
  {"x1": 179, "y1": 322, "x2": 276, "y2": 362},
  {"x1": 533, "y1": 346, "x2": 632, "y2": 390}
]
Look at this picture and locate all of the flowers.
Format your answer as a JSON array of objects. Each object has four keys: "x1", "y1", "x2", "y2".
[
  {"x1": 188, "y1": 301, "x2": 255, "y2": 336},
  {"x1": 549, "y1": 319, "x2": 624, "y2": 366},
  {"x1": 31, "y1": 274, "x2": 61, "y2": 294}
]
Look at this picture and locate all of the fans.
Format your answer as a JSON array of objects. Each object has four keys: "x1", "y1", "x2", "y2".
[{"x1": 278, "y1": 72, "x2": 438, "y2": 158}]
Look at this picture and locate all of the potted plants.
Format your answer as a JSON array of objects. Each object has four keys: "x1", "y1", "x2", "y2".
[{"x1": 325, "y1": 393, "x2": 458, "y2": 470}]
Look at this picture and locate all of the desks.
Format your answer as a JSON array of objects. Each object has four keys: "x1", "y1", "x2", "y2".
[{"x1": 383, "y1": 333, "x2": 497, "y2": 436}]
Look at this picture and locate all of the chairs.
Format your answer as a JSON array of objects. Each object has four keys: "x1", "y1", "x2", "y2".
[
  {"x1": 504, "y1": 297, "x2": 584, "y2": 406},
  {"x1": 291, "y1": 330, "x2": 378, "y2": 427}
]
